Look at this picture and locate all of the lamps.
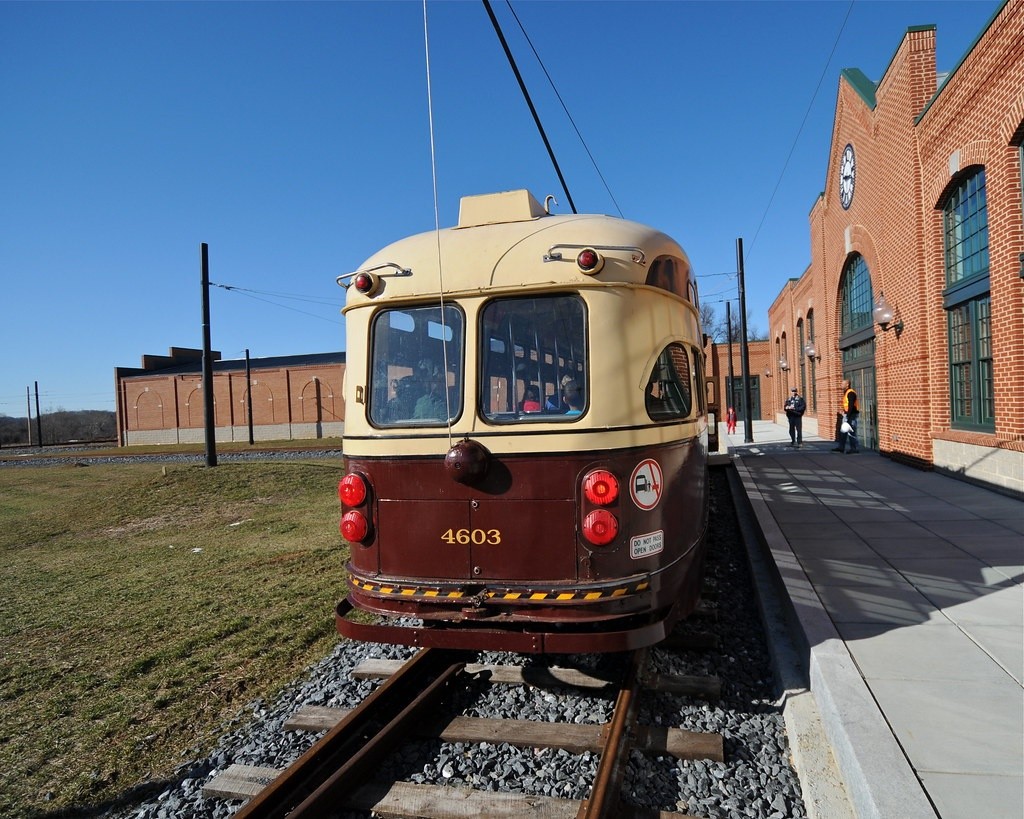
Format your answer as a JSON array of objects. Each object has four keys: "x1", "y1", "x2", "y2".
[
  {"x1": 764, "y1": 364, "x2": 773, "y2": 378},
  {"x1": 805, "y1": 337, "x2": 821, "y2": 363},
  {"x1": 872, "y1": 291, "x2": 903, "y2": 339},
  {"x1": 779, "y1": 353, "x2": 790, "y2": 372}
]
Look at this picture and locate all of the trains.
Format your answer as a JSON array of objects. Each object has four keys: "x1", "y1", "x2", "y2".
[{"x1": 334, "y1": 188, "x2": 711, "y2": 654}]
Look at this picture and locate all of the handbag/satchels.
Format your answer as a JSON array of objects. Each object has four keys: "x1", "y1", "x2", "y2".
[{"x1": 840, "y1": 414, "x2": 854, "y2": 433}]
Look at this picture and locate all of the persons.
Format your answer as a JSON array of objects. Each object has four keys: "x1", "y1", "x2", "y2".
[
  {"x1": 831, "y1": 379, "x2": 861, "y2": 454},
  {"x1": 518, "y1": 385, "x2": 540, "y2": 413},
  {"x1": 783, "y1": 387, "x2": 806, "y2": 447},
  {"x1": 386, "y1": 342, "x2": 447, "y2": 421},
  {"x1": 562, "y1": 378, "x2": 585, "y2": 416},
  {"x1": 545, "y1": 375, "x2": 574, "y2": 413},
  {"x1": 726, "y1": 407, "x2": 736, "y2": 435}
]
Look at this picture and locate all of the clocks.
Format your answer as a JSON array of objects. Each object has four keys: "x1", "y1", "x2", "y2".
[{"x1": 838, "y1": 143, "x2": 856, "y2": 210}]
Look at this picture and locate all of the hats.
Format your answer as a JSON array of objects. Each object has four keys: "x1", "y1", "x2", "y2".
[{"x1": 791, "y1": 387, "x2": 797, "y2": 392}]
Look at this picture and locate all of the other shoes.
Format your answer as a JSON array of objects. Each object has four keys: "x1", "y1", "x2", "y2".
[
  {"x1": 791, "y1": 442, "x2": 802, "y2": 448},
  {"x1": 846, "y1": 449, "x2": 860, "y2": 455},
  {"x1": 831, "y1": 448, "x2": 844, "y2": 453}
]
